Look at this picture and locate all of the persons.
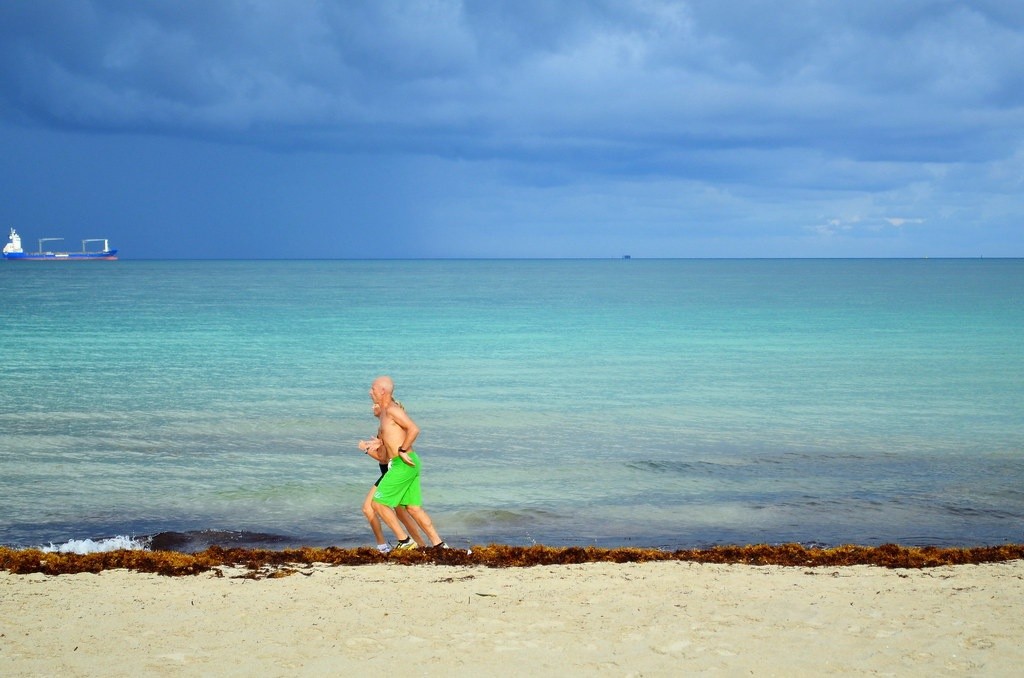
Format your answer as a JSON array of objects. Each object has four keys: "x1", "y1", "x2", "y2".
[
  {"x1": 366, "y1": 376, "x2": 450, "y2": 549},
  {"x1": 359, "y1": 397, "x2": 428, "y2": 553}
]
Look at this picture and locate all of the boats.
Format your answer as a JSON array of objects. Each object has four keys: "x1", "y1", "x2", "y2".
[{"x1": 2, "y1": 226, "x2": 121, "y2": 262}]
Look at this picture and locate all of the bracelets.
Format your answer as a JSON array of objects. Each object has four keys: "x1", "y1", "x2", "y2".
[
  {"x1": 364, "y1": 447, "x2": 370, "y2": 454},
  {"x1": 398, "y1": 446, "x2": 406, "y2": 452}
]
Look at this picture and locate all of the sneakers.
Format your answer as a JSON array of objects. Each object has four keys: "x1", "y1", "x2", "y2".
[
  {"x1": 434, "y1": 542, "x2": 449, "y2": 549},
  {"x1": 395, "y1": 536, "x2": 417, "y2": 550}
]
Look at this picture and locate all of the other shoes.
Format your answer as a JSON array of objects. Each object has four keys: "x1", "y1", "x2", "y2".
[{"x1": 376, "y1": 544, "x2": 389, "y2": 553}]
